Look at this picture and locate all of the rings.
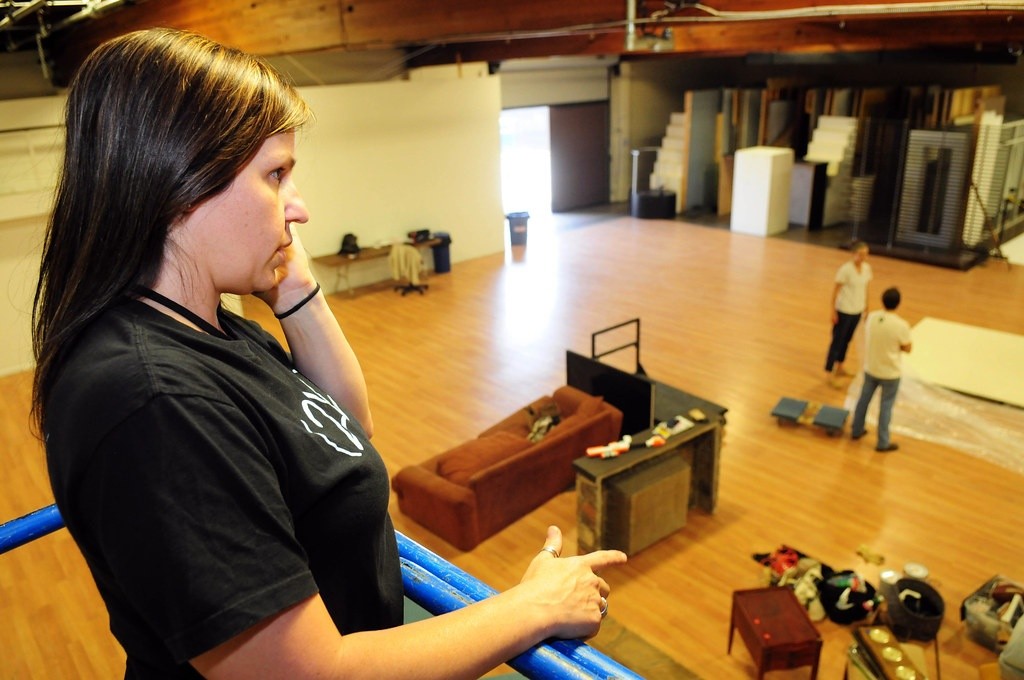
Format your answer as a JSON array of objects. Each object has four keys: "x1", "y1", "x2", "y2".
[
  {"x1": 538, "y1": 546, "x2": 558, "y2": 557},
  {"x1": 599, "y1": 596, "x2": 608, "y2": 615}
]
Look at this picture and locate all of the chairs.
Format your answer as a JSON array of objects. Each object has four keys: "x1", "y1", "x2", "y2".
[{"x1": 390, "y1": 243, "x2": 428, "y2": 296}]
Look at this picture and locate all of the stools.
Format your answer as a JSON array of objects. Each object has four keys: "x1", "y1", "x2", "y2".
[{"x1": 725, "y1": 585, "x2": 824, "y2": 680}]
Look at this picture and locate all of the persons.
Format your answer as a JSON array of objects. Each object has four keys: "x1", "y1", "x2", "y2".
[
  {"x1": 822, "y1": 240, "x2": 873, "y2": 390},
  {"x1": 28, "y1": 27, "x2": 627, "y2": 680},
  {"x1": 851, "y1": 287, "x2": 913, "y2": 451}
]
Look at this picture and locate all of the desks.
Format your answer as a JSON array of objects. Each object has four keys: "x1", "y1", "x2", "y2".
[
  {"x1": 571, "y1": 372, "x2": 729, "y2": 562},
  {"x1": 311, "y1": 235, "x2": 444, "y2": 294}
]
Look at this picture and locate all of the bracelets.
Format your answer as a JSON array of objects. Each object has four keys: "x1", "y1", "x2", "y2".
[{"x1": 276, "y1": 285, "x2": 321, "y2": 318}]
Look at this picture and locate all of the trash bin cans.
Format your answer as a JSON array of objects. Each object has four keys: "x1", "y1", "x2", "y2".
[
  {"x1": 506, "y1": 210, "x2": 530, "y2": 246},
  {"x1": 431, "y1": 232, "x2": 452, "y2": 275}
]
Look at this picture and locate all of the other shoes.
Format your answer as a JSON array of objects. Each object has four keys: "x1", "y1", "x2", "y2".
[
  {"x1": 877, "y1": 443, "x2": 898, "y2": 451},
  {"x1": 853, "y1": 429, "x2": 867, "y2": 438}
]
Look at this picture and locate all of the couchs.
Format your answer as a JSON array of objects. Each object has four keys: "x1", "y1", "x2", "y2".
[{"x1": 392, "y1": 388, "x2": 623, "y2": 552}]
